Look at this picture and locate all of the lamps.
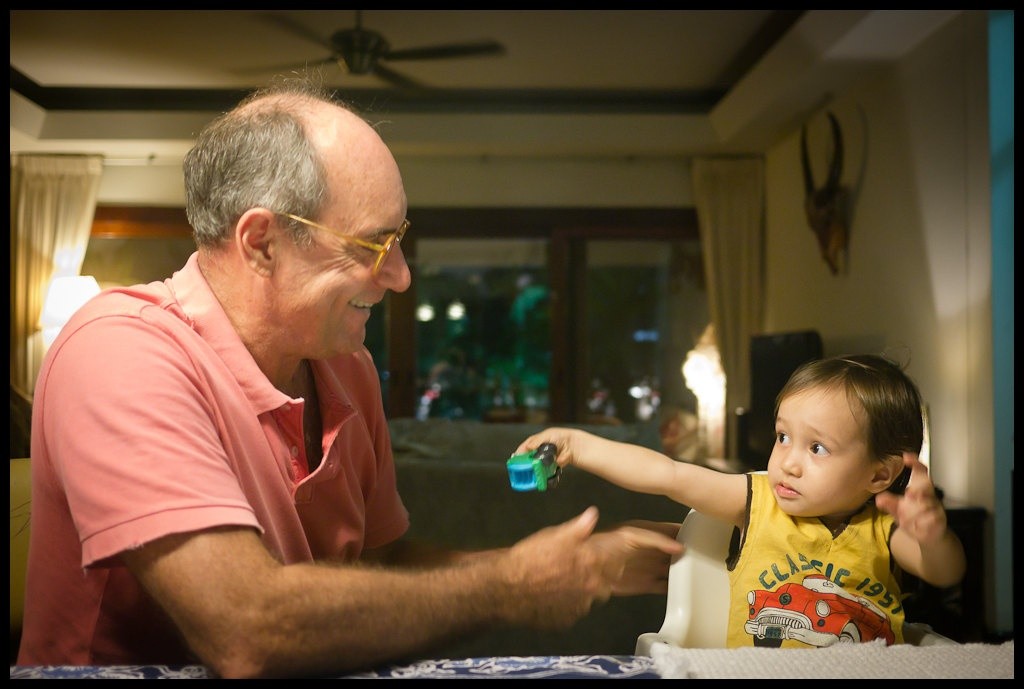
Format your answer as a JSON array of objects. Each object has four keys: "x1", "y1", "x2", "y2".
[
  {"x1": 36, "y1": 274, "x2": 103, "y2": 331},
  {"x1": 682, "y1": 315, "x2": 726, "y2": 404}
]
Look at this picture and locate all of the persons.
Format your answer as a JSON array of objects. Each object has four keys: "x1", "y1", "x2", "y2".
[
  {"x1": 12, "y1": 65, "x2": 687, "y2": 685},
  {"x1": 510, "y1": 343, "x2": 969, "y2": 650}
]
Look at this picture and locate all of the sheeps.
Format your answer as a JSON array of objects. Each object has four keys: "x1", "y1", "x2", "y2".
[{"x1": 799, "y1": 105, "x2": 852, "y2": 280}]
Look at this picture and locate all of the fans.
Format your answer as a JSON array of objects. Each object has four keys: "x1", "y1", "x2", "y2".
[{"x1": 235, "y1": 11, "x2": 504, "y2": 101}]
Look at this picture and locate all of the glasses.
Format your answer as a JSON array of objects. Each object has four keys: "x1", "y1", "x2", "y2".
[{"x1": 277, "y1": 211, "x2": 410, "y2": 278}]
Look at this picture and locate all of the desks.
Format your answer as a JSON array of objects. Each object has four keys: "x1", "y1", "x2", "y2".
[{"x1": 9, "y1": 640, "x2": 1014, "y2": 682}]
